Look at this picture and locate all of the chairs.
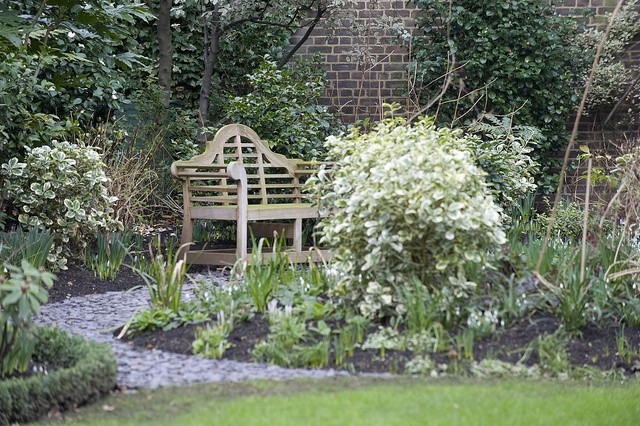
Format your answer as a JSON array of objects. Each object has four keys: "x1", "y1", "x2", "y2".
[{"x1": 171, "y1": 123, "x2": 340, "y2": 274}]
